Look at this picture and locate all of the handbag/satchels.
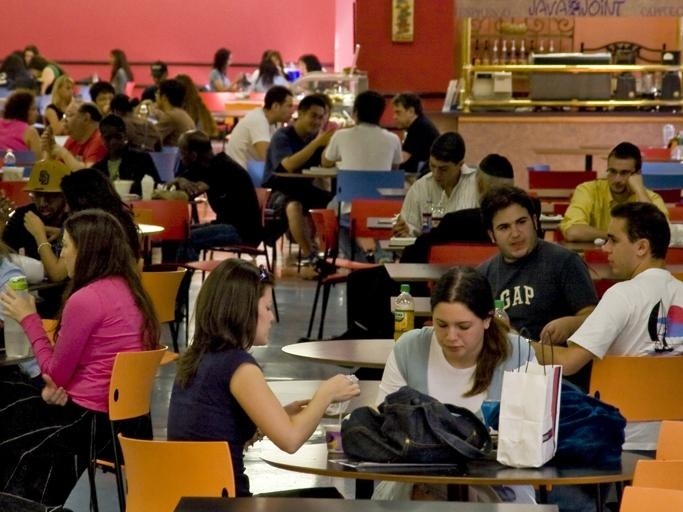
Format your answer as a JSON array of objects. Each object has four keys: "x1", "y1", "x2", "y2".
[
  {"x1": 496, "y1": 327, "x2": 563, "y2": 468},
  {"x1": 340, "y1": 386, "x2": 492, "y2": 471}
]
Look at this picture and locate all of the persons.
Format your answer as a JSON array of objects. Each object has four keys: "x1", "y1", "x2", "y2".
[
  {"x1": 1, "y1": 44, "x2": 600, "y2": 348},
  {"x1": 369, "y1": 265, "x2": 539, "y2": 503},
  {"x1": 0, "y1": 208, "x2": 162, "y2": 512},
  {"x1": 558, "y1": 142, "x2": 670, "y2": 242},
  {"x1": 529, "y1": 202, "x2": 683, "y2": 460},
  {"x1": 168, "y1": 257, "x2": 342, "y2": 498}
]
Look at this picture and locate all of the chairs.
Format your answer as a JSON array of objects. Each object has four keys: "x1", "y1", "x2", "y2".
[
  {"x1": 631, "y1": 460, "x2": 683, "y2": 489},
  {"x1": 590, "y1": 355, "x2": 683, "y2": 511},
  {"x1": 620, "y1": 485, "x2": 683, "y2": 511},
  {"x1": 657, "y1": 420, "x2": 683, "y2": 462},
  {"x1": 116, "y1": 433, "x2": 233, "y2": 512},
  {"x1": 261, "y1": 442, "x2": 653, "y2": 512},
  {"x1": 89, "y1": 346, "x2": 168, "y2": 512}
]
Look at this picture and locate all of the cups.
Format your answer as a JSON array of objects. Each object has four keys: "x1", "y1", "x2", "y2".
[{"x1": 326, "y1": 426, "x2": 345, "y2": 453}]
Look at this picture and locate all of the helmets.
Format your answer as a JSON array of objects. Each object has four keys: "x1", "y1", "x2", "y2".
[{"x1": 23, "y1": 159, "x2": 71, "y2": 192}]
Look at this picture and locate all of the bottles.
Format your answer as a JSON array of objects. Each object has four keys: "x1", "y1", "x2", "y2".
[
  {"x1": 473, "y1": 40, "x2": 554, "y2": 65},
  {"x1": 494, "y1": 299, "x2": 509, "y2": 326},
  {"x1": 431, "y1": 207, "x2": 442, "y2": 230},
  {"x1": 394, "y1": 284, "x2": 416, "y2": 340},
  {"x1": 140, "y1": 105, "x2": 147, "y2": 119},
  {"x1": 141, "y1": 175, "x2": 154, "y2": 200},
  {"x1": 422, "y1": 200, "x2": 432, "y2": 233},
  {"x1": 4, "y1": 276, "x2": 30, "y2": 358}
]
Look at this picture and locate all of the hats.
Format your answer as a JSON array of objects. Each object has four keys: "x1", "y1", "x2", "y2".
[{"x1": 479, "y1": 154, "x2": 515, "y2": 178}]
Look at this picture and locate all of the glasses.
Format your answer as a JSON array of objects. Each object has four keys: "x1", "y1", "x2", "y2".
[{"x1": 604, "y1": 168, "x2": 640, "y2": 177}]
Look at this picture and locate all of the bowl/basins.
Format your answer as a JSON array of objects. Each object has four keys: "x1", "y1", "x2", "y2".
[{"x1": 114, "y1": 180, "x2": 135, "y2": 195}]
